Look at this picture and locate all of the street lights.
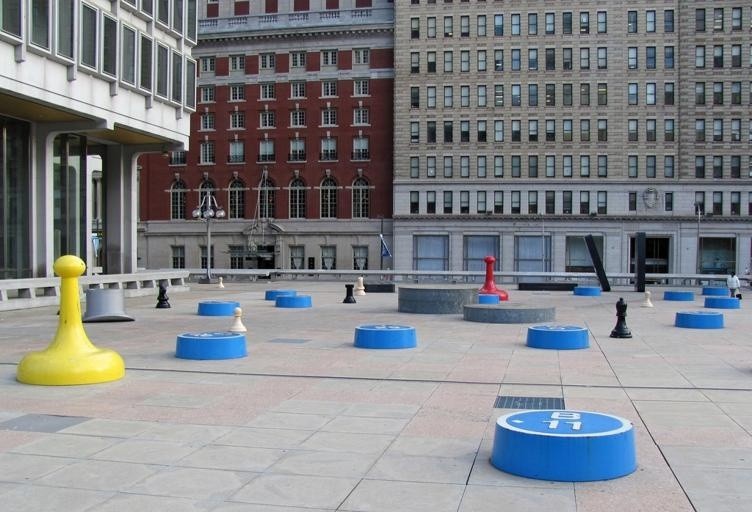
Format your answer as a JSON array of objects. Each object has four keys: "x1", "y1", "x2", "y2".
[
  {"x1": 694, "y1": 201, "x2": 700, "y2": 274},
  {"x1": 192, "y1": 195, "x2": 225, "y2": 279}
]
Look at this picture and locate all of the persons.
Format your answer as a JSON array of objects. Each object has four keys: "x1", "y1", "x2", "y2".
[{"x1": 726, "y1": 272, "x2": 740, "y2": 298}]
